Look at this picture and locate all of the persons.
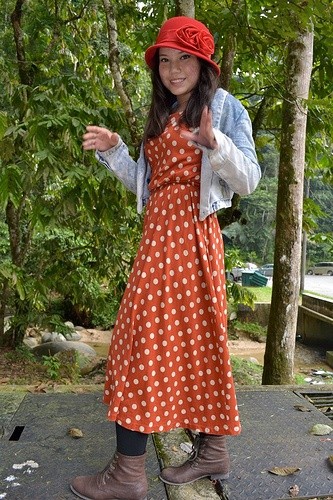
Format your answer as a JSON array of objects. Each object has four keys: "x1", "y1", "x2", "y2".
[{"x1": 70, "y1": 17, "x2": 262, "y2": 499}]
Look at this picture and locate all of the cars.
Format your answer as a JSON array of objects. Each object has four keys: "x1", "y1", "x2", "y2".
[
  {"x1": 227, "y1": 262, "x2": 259, "y2": 282},
  {"x1": 260, "y1": 264, "x2": 274, "y2": 277}
]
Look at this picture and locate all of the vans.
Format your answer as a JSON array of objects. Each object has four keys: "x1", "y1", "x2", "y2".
[{"x1": 306, "y1": 261, "x2": 333, "y2": 276}]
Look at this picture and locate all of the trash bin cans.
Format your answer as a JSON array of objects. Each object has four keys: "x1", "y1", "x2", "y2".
[{"x1": 242, "y1": 272, "x2": 268, "y2": 286}]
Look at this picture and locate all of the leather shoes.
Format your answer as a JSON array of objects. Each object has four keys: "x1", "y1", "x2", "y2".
[
  {"x1": 69, "y1": 451, "x2": 148, "y2": 500},
  {"x1": 158, "y1": 433, "x2": 229, "y2": 486}
]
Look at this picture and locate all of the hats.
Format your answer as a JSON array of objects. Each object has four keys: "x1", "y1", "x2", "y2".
[{"x1": 144, "y1": 16, "x2": 220, "y2": 80}]
